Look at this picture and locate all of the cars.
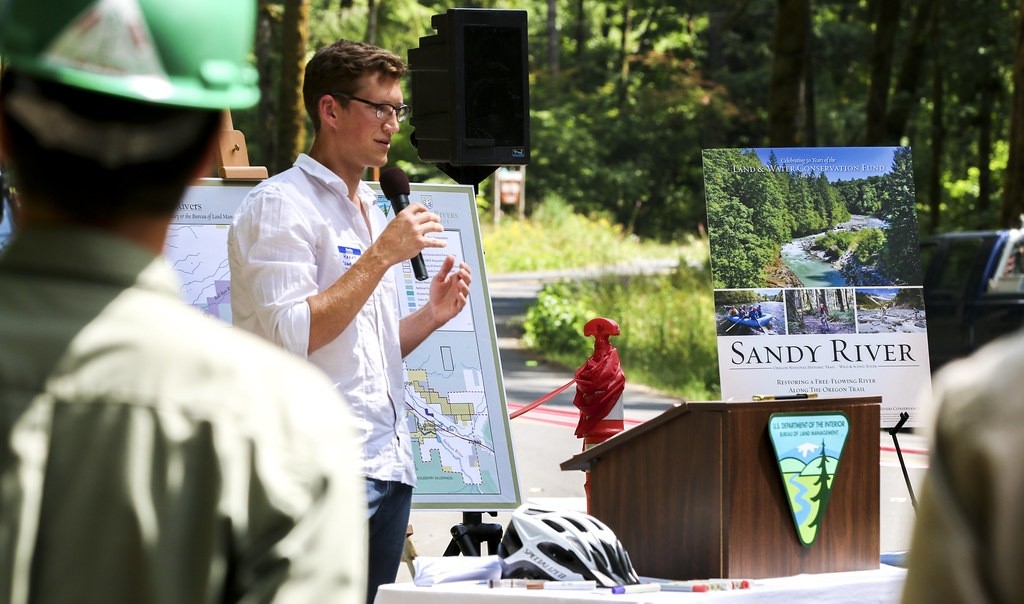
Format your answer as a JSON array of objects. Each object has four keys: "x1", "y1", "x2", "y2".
[{"x1": 918, "y1": 229, "x2": 1023, "y2": 377}]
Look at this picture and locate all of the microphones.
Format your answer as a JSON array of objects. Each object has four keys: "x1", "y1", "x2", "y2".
[{"x1": 379, "y1": 168, "x2": 428, "y2": 281}]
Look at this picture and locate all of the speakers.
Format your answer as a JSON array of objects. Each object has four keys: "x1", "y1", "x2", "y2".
[{"x1": 410, "y1": 8, "x2": 531, "y2": 165}]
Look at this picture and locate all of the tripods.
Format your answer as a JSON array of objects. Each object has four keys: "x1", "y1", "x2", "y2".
[{"x1": 443, "y1": 512, "x2": 503, "y2": 557}]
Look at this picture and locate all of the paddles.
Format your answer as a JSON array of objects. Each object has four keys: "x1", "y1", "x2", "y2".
[
  {"x1": 719, "y1": 316, "x2": 732, "y2": 325},
  {"x1": 754, "y1": 316, "x2": 768, "y2": 335},
  {"x1": 762, "y1": 313, "x2": 780, "y2": 322},
  {"x1": 725, "y1": 316, "x2": 746, "y2": 332}
]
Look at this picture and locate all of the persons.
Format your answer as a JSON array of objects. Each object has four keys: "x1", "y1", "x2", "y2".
[
  {"x1": 819, "y1": 303, "x2": 830, "y2": 329},
  {"x1": 723, "y1": 304, "x2": 763, "y2": 320},
  {"x1": 901, "y1": 328, "x2": 1024, "y2": 604},
  {"x1": 0, "y1": 0, "x2": 472, "y2": 604}
]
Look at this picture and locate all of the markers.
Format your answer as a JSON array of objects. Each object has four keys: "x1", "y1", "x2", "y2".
[
  {"x1": 613, "y1": 578, "x2": 750, "y2": 594},
  {"x1": 488, "y1": 578, "x2": 597, "y2": 594}
]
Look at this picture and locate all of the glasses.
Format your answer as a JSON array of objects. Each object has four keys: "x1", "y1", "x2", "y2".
[{"x1": 332, "y1": 93, "x2": 411, "y2": 122}]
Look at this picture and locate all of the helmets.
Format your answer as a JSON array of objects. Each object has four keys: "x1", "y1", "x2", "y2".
[
  {"x1": 498, "y1": 503, "x2": 641, "y2": 587},
  {"x1": 0, "y1": 0, "x2": 263, "y2": 110}
]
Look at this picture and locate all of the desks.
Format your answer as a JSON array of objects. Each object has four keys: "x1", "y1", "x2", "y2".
[{"x1": 374, "y1": 563, "x2": 910, "y2": 604}]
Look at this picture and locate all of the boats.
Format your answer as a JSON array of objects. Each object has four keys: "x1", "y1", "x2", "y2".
[{"x1": 727, "y1": 313, "x2": 778, "y2": 327}]
